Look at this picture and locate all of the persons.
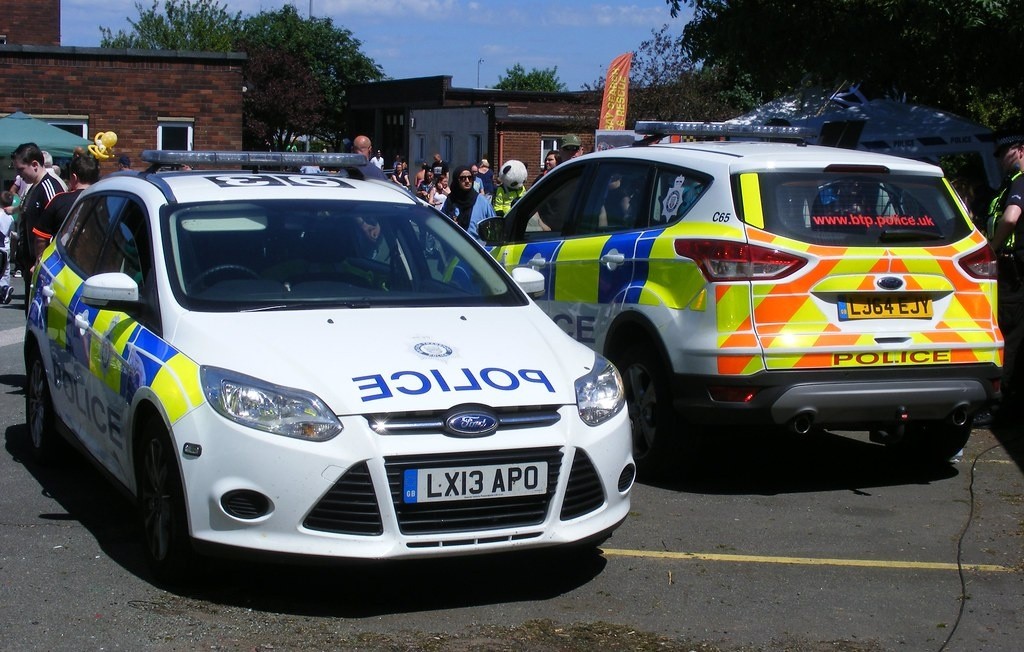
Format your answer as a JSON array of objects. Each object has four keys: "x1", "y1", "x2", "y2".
[
  {"x1": 286, "y1": 134, "x2": 638, "y2": 270},
  {"x1": 0, "y1": 143, "x2": 192, "y2": 320},
  {"x1": 972, "y1": 121, "x2": 1024, "y2": 428},
  {"x1": 437, "y1": 165, "x2": 497, "y2": 292}
]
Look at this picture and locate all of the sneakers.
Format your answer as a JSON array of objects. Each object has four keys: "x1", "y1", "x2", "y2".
[
  {"x1": 13, "y1": 270, "x2": 22, "y2": 278},
  {"x1": 0, "y1": 285, "x2": 14, "y2": 305}
]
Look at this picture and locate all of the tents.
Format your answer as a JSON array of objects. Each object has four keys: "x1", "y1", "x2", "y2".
[{"x1": 724, "y1": 83, "x2": 1005, "y2": 194}]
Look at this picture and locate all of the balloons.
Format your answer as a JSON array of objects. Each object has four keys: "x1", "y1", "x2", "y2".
[
  {"x1": 7, "y1": 194, "x2": 21, "y2": 220},
  {"x1": 88, "y1": 131, "x2": 117, "y2": 159}
]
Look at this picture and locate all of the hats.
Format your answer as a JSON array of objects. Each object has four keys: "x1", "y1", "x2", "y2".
[
  {"x1": 560, "y1": 134, "x2": 582, "y2": 148},
  {"x1": 975, "y1": 117, "x2": 1024, "y2": 143}
]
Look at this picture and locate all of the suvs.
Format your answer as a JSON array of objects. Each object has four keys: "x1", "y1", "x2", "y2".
[
  {"x1": 477, "y1": 118, "x2": 1006, "y2": 475},
  {"x1": 25, "y1": 150, "x2": 637, "y2": 587}
]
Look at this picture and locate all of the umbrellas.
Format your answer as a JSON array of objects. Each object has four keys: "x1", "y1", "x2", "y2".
[{"x1": 0, "y1": 111, "x2": 97, "y2": 158}]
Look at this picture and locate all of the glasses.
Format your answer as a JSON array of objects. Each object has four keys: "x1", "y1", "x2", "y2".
[
  {"x1": 562, "y1": 145, "x2": 580, "y2": 152},
  {"x1": 458, "y1": 176, "x2": 476, "y2": 182},
  {"x1": 992, "y1": 140, "x2": 1024, "y2": 159}
]
